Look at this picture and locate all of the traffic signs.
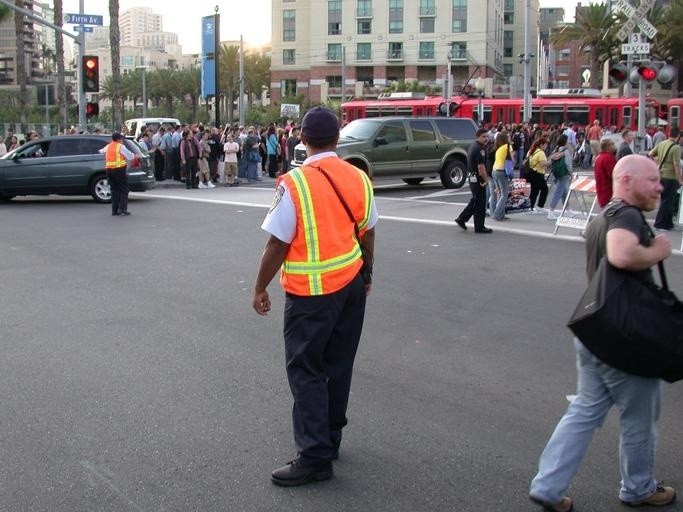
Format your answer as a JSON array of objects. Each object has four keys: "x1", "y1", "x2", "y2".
[{"x1": 61, "y1": 13, "x2": 104, "y2": 25}]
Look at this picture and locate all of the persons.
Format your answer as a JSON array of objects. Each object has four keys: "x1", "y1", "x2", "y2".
[
  {"x1": 0, "y1": 125, "x2": 111, "y2": 158},
  {"x1": 454, "y1": 129, "x2": 493, "y2": 234},
  {"x1": 251, "y1": 105, "x2": 377, "y2": 487},
  {"x1": 479, "y1": 120, "x2": 683, "y2": 233},
  {"x1": 105, "y1": 131, "x2": 135, "y2": 216},
  {"x1": 528, "y1": 148, "x2": 683, "y2": 512},
  {"x1": 119, "y1": 118, "x2": 300, "y2": 191}
]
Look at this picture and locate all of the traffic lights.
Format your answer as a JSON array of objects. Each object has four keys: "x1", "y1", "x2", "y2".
[
  {"x1": 86, "y1": 103, "x2": 100, "y2": 116},
  {"x1": 608, "y1": 58, "x2": 677, "y2": 86},
  {"x1": 83, "y1": 55, "x2": 99, "y2": 91}
]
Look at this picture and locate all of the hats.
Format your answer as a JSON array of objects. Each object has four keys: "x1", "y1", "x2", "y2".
[{"x1": 300, "y1": 105, "x2": 340, "y2": 139}]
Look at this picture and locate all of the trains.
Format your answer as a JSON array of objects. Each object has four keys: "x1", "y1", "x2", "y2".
[{"x1": 339, "y1": 91, "x2": 683, "y2": 136}]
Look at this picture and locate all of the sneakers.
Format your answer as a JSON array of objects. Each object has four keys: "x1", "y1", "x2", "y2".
[
  {"x1": 270, "y1": 454, "x2": 332, "y2": 486},
  {"x1": 186, "y1": 180, "x2": 244, "y2": 190},
  {"x1": 111, "y1": 210, "x2": 132, "y2": 216},
  {"x1": 485, "y1": 212, "x2": 511, "y2": 221},
  {"x1": 453, "y1": 217, "x2": 493, "y2": 234},
  {"x1": 527, "y1": 490, "x2": 573, "y2": 512},
  {"x1": 529, "y1": 205, "x2": 579, "y2": 220},
  {"x1": 619, "y1": 479, "x2": 677, "y2": 507}
]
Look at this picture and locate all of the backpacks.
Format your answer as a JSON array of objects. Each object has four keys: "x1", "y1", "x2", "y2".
[
  {"x1": 551, "y1": 146, "x2": 570, "y2": 178},
  {"x1": 519, "y1": 149, "x2": 543, "y2": 179}
]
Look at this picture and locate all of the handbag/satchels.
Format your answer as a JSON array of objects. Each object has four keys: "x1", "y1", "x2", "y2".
[
  {"x1": 504, "y1": 157, "x2": 515, "y2": 180},
  {"x1": 566, "y1": 206, "x2": 683, "y2": 382}
]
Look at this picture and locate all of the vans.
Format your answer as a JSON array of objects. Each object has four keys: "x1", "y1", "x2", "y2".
[
  {"x1": 120, "y1": 117, "x2": 183, "y2": 140},
  {"x1": 0, "y1": 134, "x2": 157, "y2": 205}
]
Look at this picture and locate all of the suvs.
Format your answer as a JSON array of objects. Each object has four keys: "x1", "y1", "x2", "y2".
[{"x1": 289, "y1": 116, "x2": 481, "y2": 189}]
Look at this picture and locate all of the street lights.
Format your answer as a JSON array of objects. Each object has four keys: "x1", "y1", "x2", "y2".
[
  {"x1": 517, "y1": 52, "x2": 535, "y2": 123},
  {"x1": 135, "y1": 65, "x2": 149, "y2": 118},
  {"x1": 232, "y1": 75, "x2": 246, "y2": 125}
]
